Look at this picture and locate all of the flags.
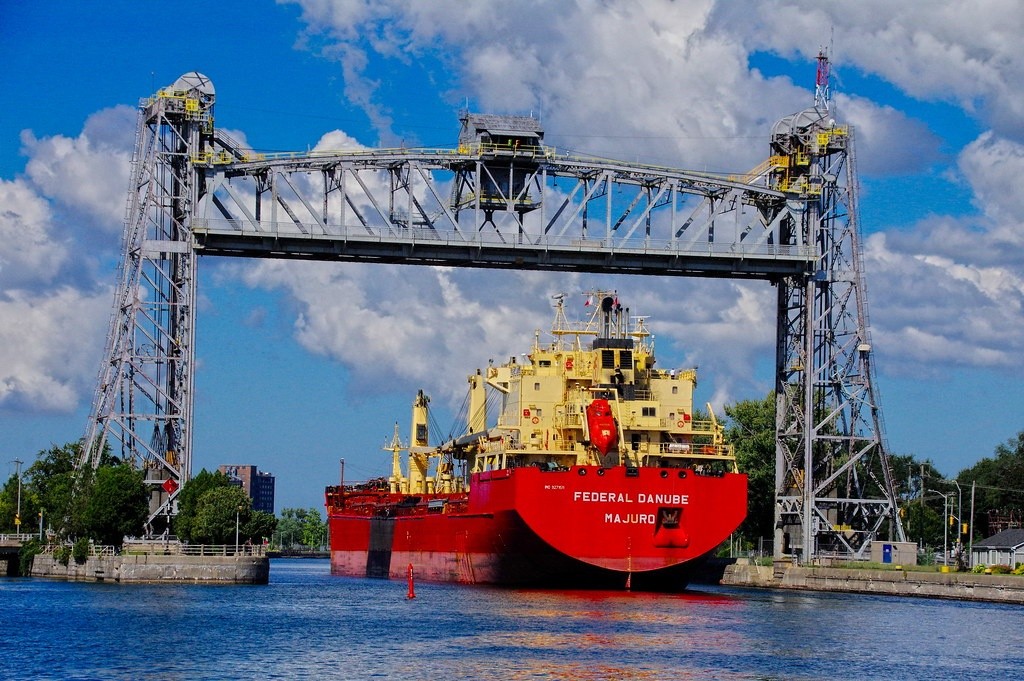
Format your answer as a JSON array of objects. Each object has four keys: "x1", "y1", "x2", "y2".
[
  {"x1": 612, "y1": 297, "x2": 617, "y2": 307},
  {"x1": 584, "y1": 294, "x2": 594, "y2": 307}
]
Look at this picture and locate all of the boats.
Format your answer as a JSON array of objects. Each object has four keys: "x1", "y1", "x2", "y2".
[{"x1": 324, "y1": 288, "x2": 748, "y2": 594}]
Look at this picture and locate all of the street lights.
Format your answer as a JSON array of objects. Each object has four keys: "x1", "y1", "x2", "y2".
[
  {"x1": 928, "y1": 489, "x2": 947, "y2": 566},
  {"x1": 948, "y1": 480, "x2": 962, "y2": 544},
  {"x1": 235, "y1": 505, "x2": 243, "y2": 552}
]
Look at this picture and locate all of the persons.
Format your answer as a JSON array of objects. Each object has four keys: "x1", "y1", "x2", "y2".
[{"x1": 245, "y1": 536, "x2": 269, "y2": 556}]
